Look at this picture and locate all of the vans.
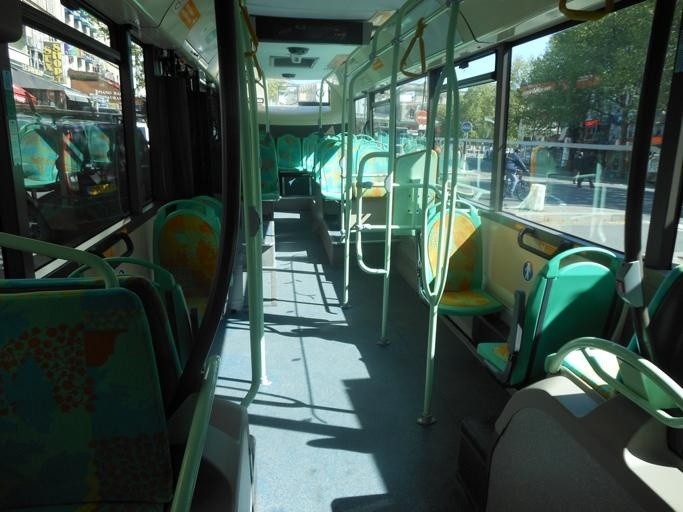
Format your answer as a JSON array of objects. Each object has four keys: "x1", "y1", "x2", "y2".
[{"x1": 16, "y1": 108, "x2": 151, "y2": 244}]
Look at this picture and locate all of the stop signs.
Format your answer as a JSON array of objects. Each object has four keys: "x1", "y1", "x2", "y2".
[{"x1": 414, "y1": 110, "x2": 428, "y2": 125}]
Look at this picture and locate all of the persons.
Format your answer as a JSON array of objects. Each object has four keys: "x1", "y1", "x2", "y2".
[
  {"x1": 504, "y1": 147, "x2": 530, "y2": 198},
  {"x1": 577, "y1": 149, "x2": 605, "y2": 189}
]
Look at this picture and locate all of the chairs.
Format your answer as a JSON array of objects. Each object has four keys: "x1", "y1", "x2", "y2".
[
  {"x1": 457, "y1": 246, "x2": 621, "y2": 388},
  {"x1": 413, "y1": 198, "x2": 503, "y2": 317},
  {"x1": 2, "y1": 195, "x2": 227, "y2": 512},
  {"x1": 542, "y1": 262, "x2": 683, "y2": 426},
  {"x1": 14, "y1": 120, "x2": 150, "y2": 192},
  {"x1": 256, "y1": 129, "x2": 442, "y2": 239}
]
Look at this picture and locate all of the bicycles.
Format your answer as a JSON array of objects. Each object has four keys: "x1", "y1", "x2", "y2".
[{"x1": 503, "y1": 169, "x2": 531, "y2": 201}]
